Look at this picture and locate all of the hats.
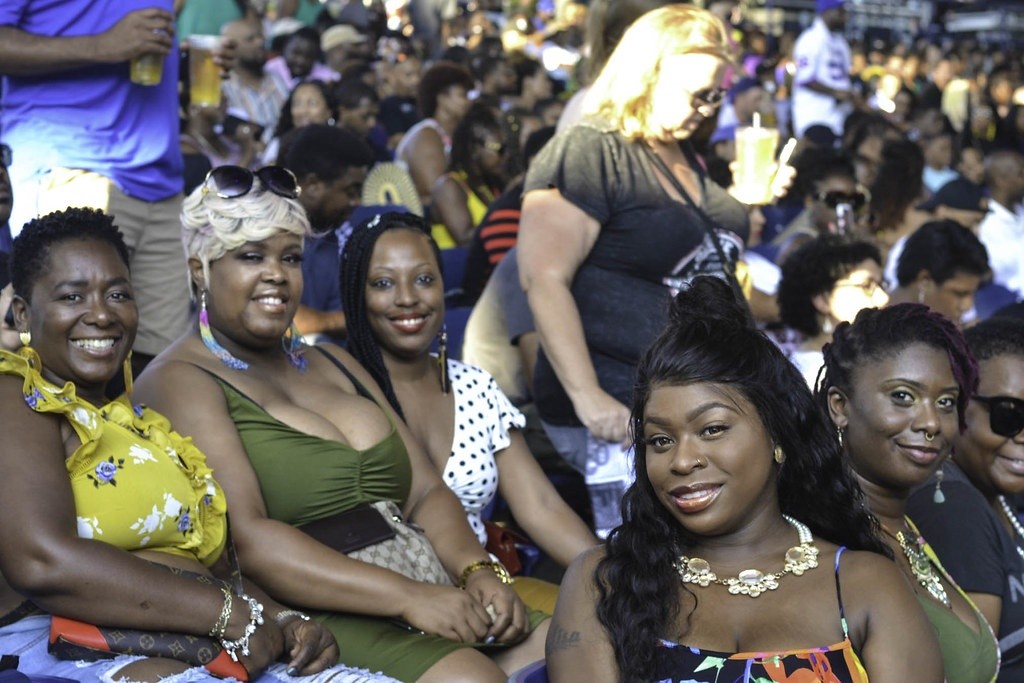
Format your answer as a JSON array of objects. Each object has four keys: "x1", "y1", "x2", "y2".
[
  {"x1": 320, "y1": 23, "x2": 366, "y2": 52},
  {"x1": 913, "y1": 176, "x2": 991, "y2": 212},
  {"x1": 815, "y1": 0, "x2": 845, "y2": 14}
]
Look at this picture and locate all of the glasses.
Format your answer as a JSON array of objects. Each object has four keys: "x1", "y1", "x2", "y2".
[
  {"x1": 202, "y1": 166, "x2": 302, "y2": 201},
  {"x1": 661, "y1": 71, "x2": 727, "y2": 102},
  {"x1": 969, "y1": 394, "x2": 1024, "y2": 438}
]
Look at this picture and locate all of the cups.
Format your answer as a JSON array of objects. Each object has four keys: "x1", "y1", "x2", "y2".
[
  {"x1": 130, "y1": 16, "x2": 168, "y2": 85},
  {"x1": 734, "y1": 125, "x2": 779, "y2": 204},
  {"x1": 188, "y1": 33, "x2": 226, "y2": 107}
]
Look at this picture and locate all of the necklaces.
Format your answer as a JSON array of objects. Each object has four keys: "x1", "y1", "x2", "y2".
[
  {"x1": 869, "y1": 515, "x2": 952, "y2": 610},
  {"x1": 998, "y1": 494, "x2": 1024, "y2": 560},
  {"x1": 670, "y1": 514, "x2": 819, "y2": 598}
]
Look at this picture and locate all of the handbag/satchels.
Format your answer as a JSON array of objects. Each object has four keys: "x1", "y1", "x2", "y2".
[
  {"x1": 48, "y1": 558, "x2": 248, "y2": 683},
  {"x1": 483, "y1": 517, "x2": 531, "y2": 576},
  {"x1": 287, "y1": 498, "x2": 456, "y2": 588}
]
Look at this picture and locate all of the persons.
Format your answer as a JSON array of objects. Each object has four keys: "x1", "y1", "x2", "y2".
[
  {"x1": 128, "y1": 166, "x2": 553, "y2": 683},
  {"x1": 905, "y1": 315, "x2": 1024, "y2": 683},
  {"x1": 545, "y1": 277, "x2": 942, "y2": 683},
  {"x1": 813, "y1": 303, "x2": 1001, "y2": 683},
  {"x1": 0, "y1": 206, "x2": 404, "y2": 683},
  {"x1": 339, "y1": 212, "x2": 603, "y2": 616},
  {"x1": 178, "y1": 0, "x2": 1024, "y2": 588},
  {"x1": 0, "y1": 0, "x2": 189, "y2": 402}
]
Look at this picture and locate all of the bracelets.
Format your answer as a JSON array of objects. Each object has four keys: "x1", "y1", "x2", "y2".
[
  {"x1": 219, "y1": 593, "x2": 264, "y2": 661},
  {"x1": 275, "y1": 610, "x2": 310, "y2": 620},
  {"x1": 209, "y1": 580, "x2": 233, "y2": 641},
  {"x1": 458, "y1": 560, "x2": 512, "y2": 589}
]
